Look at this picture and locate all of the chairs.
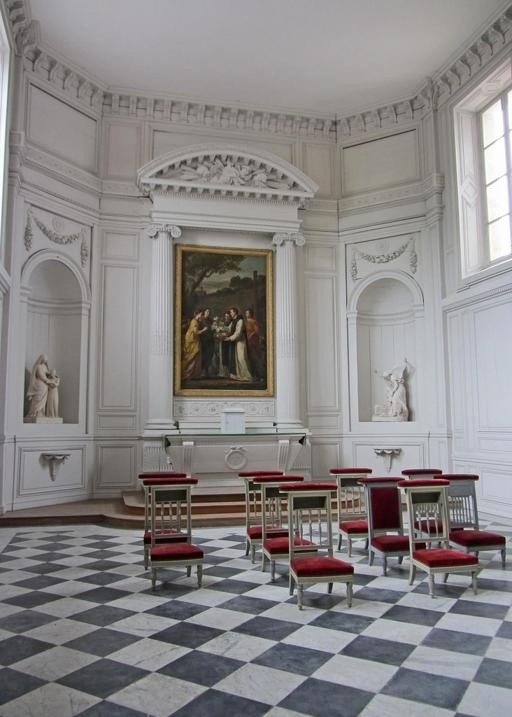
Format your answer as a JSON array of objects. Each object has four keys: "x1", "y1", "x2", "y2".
[
  {"x1": 329, "y1": 468, "x2": 372, "y2": 557},
  {"x1": 356, "y1": 477, "x2": 426, "y2": 577},
  {"x1": 398, "y1": 479, "x2": 479, "y2": 599},
  {"x1": 238, "y1": 471, "x2": 283, "y2": 564},
  {"x1": 139, "y1": 472, "x2": 186, "y2": 570},
  {"x1": 433, "y1": 474, "x2": 506, "y2": 571},
  {"x1": 253, "y1": 475, "x2": 317, "y2": 583},
  {"x1": 279, "y1": 483, "x2": 354, "y2": 611},
  {"x1": 143, "y1": 479, "x2": 203, "y2": 591},
  {"x1": 402, "y1": 469, "x2": 463, "y2": 548}
]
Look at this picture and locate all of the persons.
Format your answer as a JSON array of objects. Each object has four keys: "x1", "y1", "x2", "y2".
[
  {"x1": 382, "y1": 368, "x2": 409, "y2": 417},
  {"x1": 182, "y1": 305, "x2": 266, "y2": 384},
  {"x1": 25, "y1": 352, "x2": 59, "y2": 418},
  {"x1": 46, "y1": 368, "x2": 60, "y2": 417}
]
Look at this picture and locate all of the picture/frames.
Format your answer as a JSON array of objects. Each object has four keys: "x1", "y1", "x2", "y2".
[{"x1": 173, "y1": 243, "x2": 275, "y2": 397}]
